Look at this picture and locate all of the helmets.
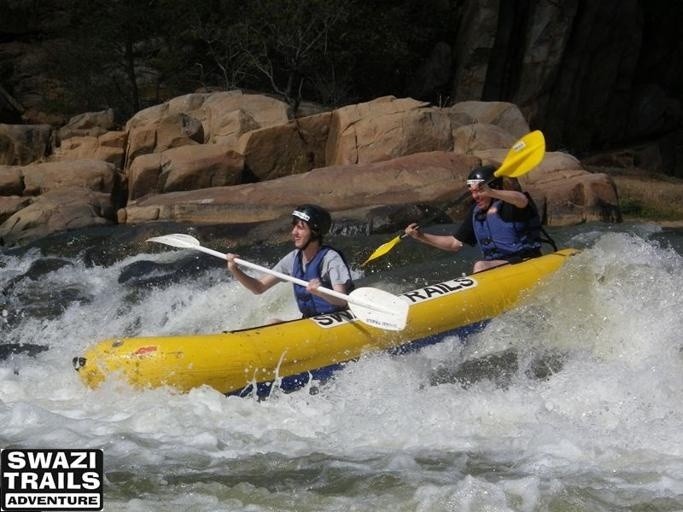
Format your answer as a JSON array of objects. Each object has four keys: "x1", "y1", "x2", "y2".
[
  {"x1": 466, "y1": 165, "x2": 504, "y2": 189},
  {"x1": 292, "y1": 203, "x2": 333, "y2": 235}
]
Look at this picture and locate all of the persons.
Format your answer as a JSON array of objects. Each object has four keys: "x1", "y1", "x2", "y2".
[
  {"x1": 405, "y1": 165, "x2": 543, "y2": 275},
  {"x1": 226, "y1": 204, "x2": 356, "y2": 324}
]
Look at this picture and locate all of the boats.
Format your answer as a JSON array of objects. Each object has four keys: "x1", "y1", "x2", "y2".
[{"x1": 72, "y1": 248, "x2": 595, "y2": 412}]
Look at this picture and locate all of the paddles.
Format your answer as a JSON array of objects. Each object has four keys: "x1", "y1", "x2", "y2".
[
  {"x1": 361, "y1": 130, "x2": 546, "y2": 267},
  {"x1": 145, "y1": 233, "x2": 408, "y2": 332}
]
